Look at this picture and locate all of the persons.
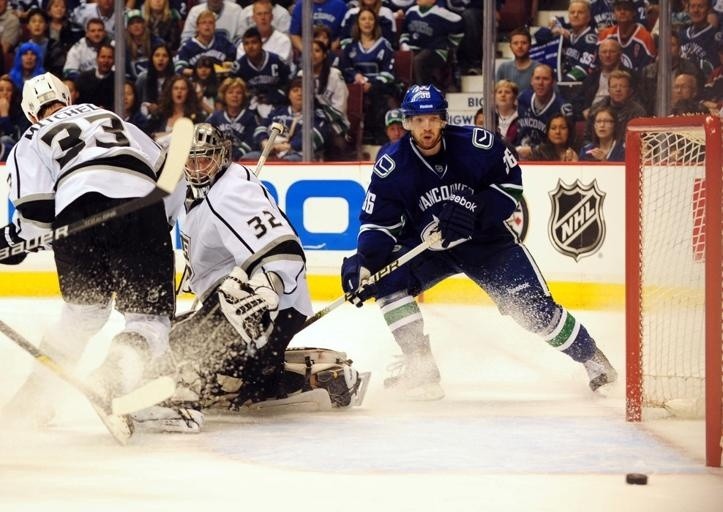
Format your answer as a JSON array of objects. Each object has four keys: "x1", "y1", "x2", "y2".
[
  {"x1": 0, "y1": 72, "x2": 204, "y2": 441},
  {"x1": 341, "y1": 85, "x2": 618, "y2": 392},
  {"x1": 163, "y1": 122, "x2": 361, "y2": 408},
  {"x1": 0, "y1": 2, "x2": 723, "y2": 165}
]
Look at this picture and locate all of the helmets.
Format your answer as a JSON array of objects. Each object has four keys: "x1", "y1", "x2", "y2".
[
  {"x1": 19, "y1": 71, "x2": 73, "y2": 125},
  {"x1": 183, "y1": 119, "x2": 232, "y2": 189},
  {"x1": 397, "y1": 80, "x2": 451, "y2": 118}
]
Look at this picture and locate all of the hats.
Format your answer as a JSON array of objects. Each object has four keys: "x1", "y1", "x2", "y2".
[{"x1": 383, "y1": 108, "x2": 403, "y2": 127}]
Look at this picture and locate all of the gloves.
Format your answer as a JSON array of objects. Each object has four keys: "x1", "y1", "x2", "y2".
[
  {"x1": 337, "y1": 251, "x2": 379, "y2": 308},
  {"x1": 0, "y1": 221, "x2": 31, "y2": 265},
  {"x1": 433, "y1": 201, "x2": 477, "y2": 248}
]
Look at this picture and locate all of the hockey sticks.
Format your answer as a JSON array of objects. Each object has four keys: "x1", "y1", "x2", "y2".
[
  {"x1": 1, "y1": 117, "x2": 195, "y2": 261},
  {"x1": 1, "y1": 321, "x2": 175, "y2": 416}
]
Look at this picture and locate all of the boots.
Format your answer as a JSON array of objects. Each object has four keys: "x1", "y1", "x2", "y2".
[
  {"x1": 378, "y1": 333, "x2": 443, "y2": 391},
  {"x1": 582, "y1": 348, "x2": 619, "y2": 394},
  {"x1": 305, "y1": 363, "x2": 364, "y2": 411},
  {"x1": 120, "y1": 382, "x2": 206, "y2": 435}
]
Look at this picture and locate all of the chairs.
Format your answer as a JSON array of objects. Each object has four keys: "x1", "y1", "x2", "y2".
[
  {"x1": 499, "y1": 0, "x2": 537, "y2": 33},
  {"x1": 574, "y1": 121, "x2": 585, "y2": 140},
  {"x1": 341, "y1": 82, "x2": 366, "y2": 162},
  {"x1": 393, "y1": 49, "x2": 414, "y2": 91}
]
